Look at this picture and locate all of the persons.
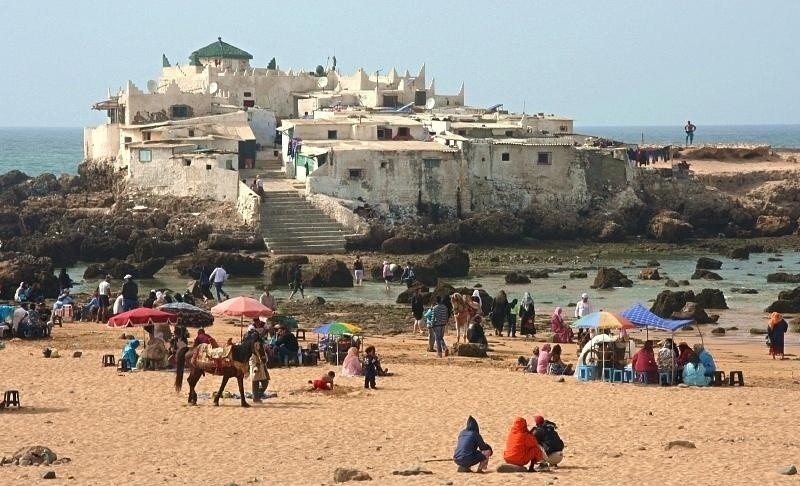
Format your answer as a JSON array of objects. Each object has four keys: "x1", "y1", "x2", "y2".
[
  {"x1": 240, "y1": 175, "x2": 266, "y2": 206},
  {"x1": 453, "y1": 414, "x2": 566, "y2": 473},
  {"x1": 684, "y1": 122, "x2": 697, "y2": 146},
  {"x1": 766, "y1": 310, "x2": 790, "y2": 362}
]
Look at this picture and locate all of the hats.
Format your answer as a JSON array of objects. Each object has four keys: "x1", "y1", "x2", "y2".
[
  {"x1": 675, "y1": 342, "x2": 686, "y2": 348},
  {"x1": 657, "y1": 339, "x2": 665, "y2": 345},
  {"x1": 105, "y1": 274, "x2": 114, "y2": 281},
  {"x1": 383, "y1": 260, "x2": 388, "y2": 264},
  {"x1": 582, "y1": 292, "x2": 588, "y2": 299}
]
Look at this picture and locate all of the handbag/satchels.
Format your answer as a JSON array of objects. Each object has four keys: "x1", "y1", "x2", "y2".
[{"x1": 390, "y1": 264, "x2": 398, "y2": 272}]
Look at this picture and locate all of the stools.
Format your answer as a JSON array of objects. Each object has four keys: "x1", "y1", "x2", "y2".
[
  {"x1": 265, "y1": 328, "x2": 359, "y2": 369},
  {"x1": 101, "y1": 354, "x2": 115, "y2": 367},
  {"x1": 3, "y1": 389, "x2": 21, "y2": 409},
  {"x1": 116, "y1": 358, "x2": 132, "y2": 373},
  {"x1": 51, "y1": 314, "x2": 63, "y2": 327},
  {"x1": 576, "y1": 360, "x2": 744, "y2": 385}
]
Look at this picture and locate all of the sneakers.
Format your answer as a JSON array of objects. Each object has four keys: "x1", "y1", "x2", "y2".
[
  {"x1": 533, "y1": 464, "x2": 549, "y2": 471},
  {"x1": 495, "y1": 332, "x2": 536, "y2": 338},
  {"x1": 549, "y1": 463, "x2": 558, "y2": 469},
  {"x1": 429, "y1": 348, "x2": 450, "y2": 358}
]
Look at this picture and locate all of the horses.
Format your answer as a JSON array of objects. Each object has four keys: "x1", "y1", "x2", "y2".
[
  {"x1": 449, "y1": 292, "x2": 475, "y2": 344},
  {"x1": 173, "y1": 330, "x2": 266, "y2": 407}
]
